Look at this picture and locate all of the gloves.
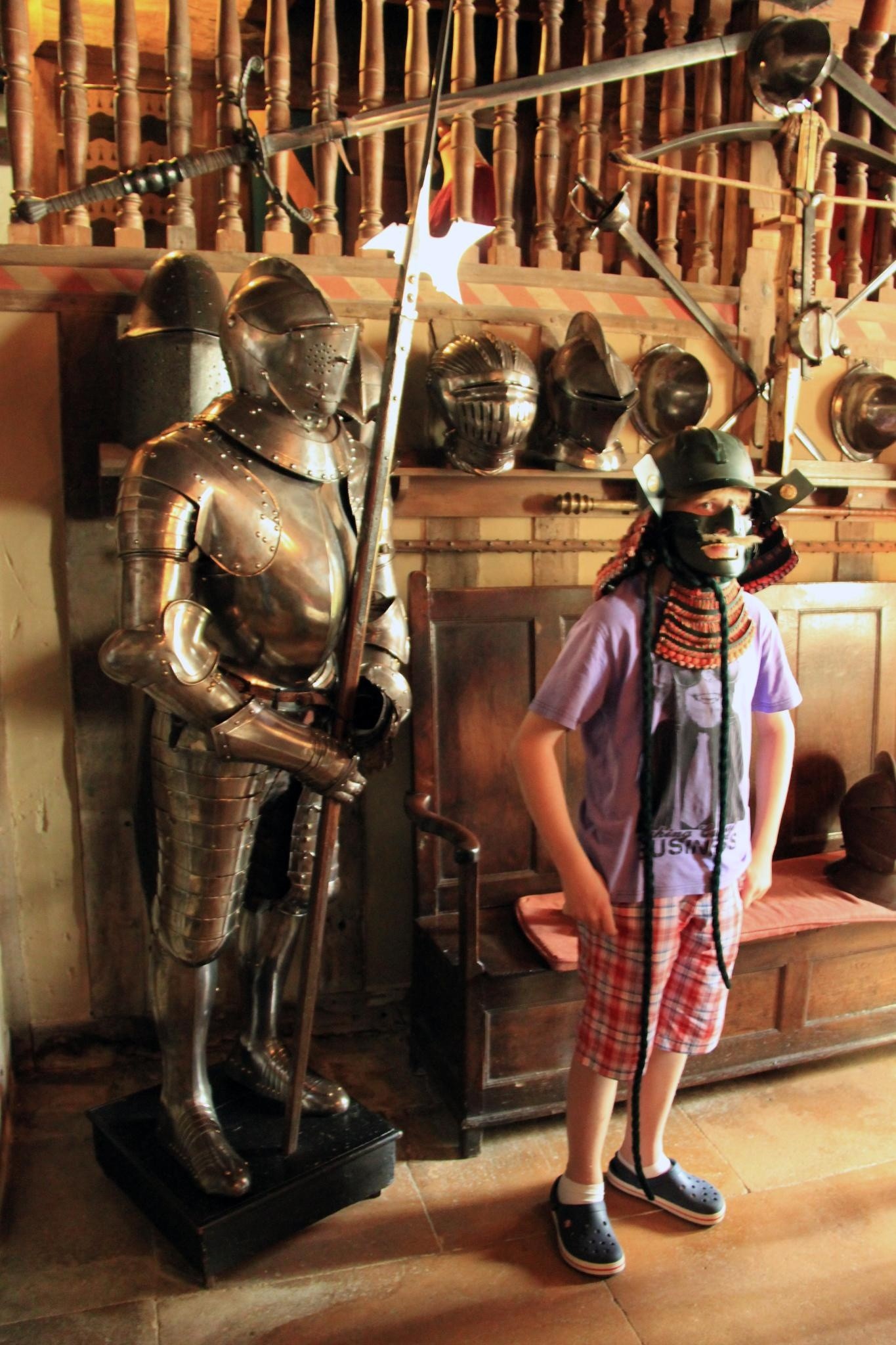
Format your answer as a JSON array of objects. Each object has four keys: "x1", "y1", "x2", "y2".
[{"x1": 210, "y1": 695, "x2": 369, "y2": 802}]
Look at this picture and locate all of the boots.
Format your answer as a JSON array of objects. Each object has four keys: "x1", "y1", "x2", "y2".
[
  {"x1": 146, "y1": 930, "x2": 254, "y2": 1198},
  {"x1": 226, "y1": 898, "x2": 350, "y2": 1117}
]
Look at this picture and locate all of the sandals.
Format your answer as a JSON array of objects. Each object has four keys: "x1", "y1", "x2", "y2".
[
  {"x1": 549, "y1": 1175, "x2": 626, "y2": 1276},
  {"x1": 606, "y1": 1149, "x2": 726, "y2": 1226}
]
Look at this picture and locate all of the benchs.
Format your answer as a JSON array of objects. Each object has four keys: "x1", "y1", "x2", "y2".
[{"x1": 406, "y1": 568, "x2": 896, "y2": 1157}]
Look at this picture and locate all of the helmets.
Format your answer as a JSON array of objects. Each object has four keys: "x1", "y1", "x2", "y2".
[
  {"x1": 837, "y1": 749, "x2": 896, "y2": 876},
  {"x1": 118, "y1": 247, "x2": 226, "y2": 337},
  {"x1": 636, "y1": 426, "x2": 772, "y2": 512},
  {"x1": 533, "y1": 310, "x2": 639, "y2": 474},
  {"x1": 629, "y1": 343, "x2": 713, "y2": 446},
  {"x1": 829, "y1": 363, "x2": 896, "y2": 465},
  {"x1": 217, "y1": 256, "x2": 358, "y2": 426},
  {"x1": 335, "y1": 339, "x2": 386, "y2": 426},
  {"x1": 423, "y1": 327, "x2": 540, "y2": 478}
]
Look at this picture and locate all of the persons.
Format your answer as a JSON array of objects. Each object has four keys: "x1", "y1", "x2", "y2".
[
  {"x1": 508, "y1": 429, "x2": 807, "y2": 1283},
  {"x1": 96, "y1": 256, "x2": 411, "y2": 1207}
]
